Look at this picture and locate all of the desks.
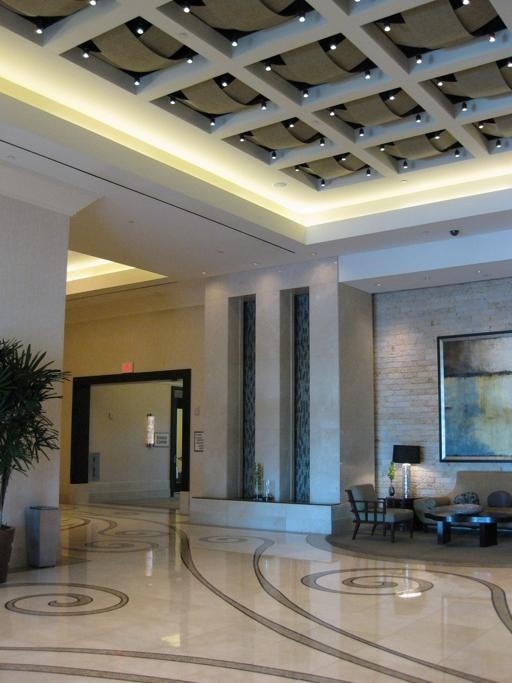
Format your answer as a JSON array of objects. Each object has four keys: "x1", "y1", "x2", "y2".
[{"x1": 384, "y1": 497, "x2": 419, "y2": 531}]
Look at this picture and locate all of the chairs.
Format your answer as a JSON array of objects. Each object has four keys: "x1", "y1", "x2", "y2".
[{"x1": 344, "y1": 482, "x2": 415, "y2": 542}]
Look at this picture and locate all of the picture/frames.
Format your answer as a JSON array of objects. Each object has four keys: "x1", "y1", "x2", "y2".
[{"x1": 437, "y1": 328, "x2": 512, "y2": 463}]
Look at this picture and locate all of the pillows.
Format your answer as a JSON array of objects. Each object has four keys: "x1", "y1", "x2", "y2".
[
  {"x1": 487, "y1": 490, "x2": 511, "y2": 509},
  {"x1": 452, "y1": 490, "x2": 480, "y2": 505}
]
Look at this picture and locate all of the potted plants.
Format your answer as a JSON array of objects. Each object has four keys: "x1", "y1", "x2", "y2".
[
  {"x1": 0, "y1": 336, "x2": 75, "y2": 588},
  {"x1": 388, "y1": 460, "x2": 397, "y2": 496}
]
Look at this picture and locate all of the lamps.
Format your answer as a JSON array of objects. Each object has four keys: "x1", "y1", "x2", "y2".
[
  {"x1": 392, "y1": 443, "x2": 421, "y2": 499},
  {"x1": 146, "y1": 411, "x2": 155, "y2": 448}
]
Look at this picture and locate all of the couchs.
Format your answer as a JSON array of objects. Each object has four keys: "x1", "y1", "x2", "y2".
[{"x1": 417, "y1": 469, "x2": 512, "y2": 533}]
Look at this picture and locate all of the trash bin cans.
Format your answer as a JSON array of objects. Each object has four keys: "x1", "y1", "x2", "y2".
[{"x1": 25, "y1": 506, "x2": 59, "y2": 568}]
